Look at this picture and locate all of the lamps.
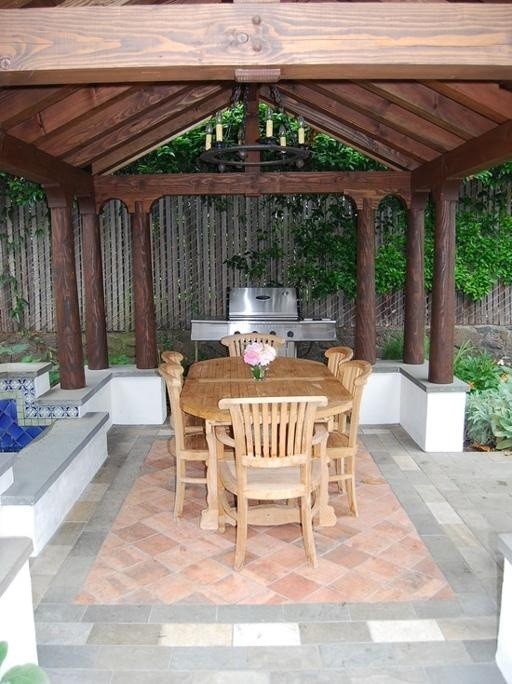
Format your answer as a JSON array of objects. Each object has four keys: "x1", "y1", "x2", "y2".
[{"x1": 200, "y1": 79, "x2": 310, "y2": 172}]
